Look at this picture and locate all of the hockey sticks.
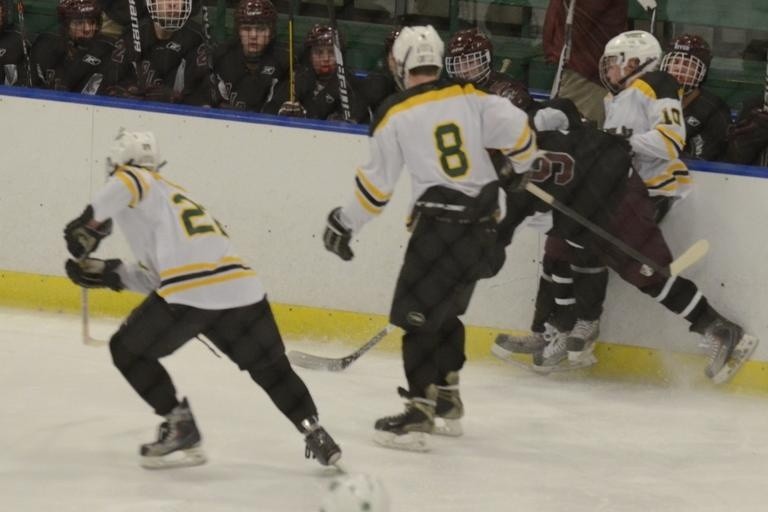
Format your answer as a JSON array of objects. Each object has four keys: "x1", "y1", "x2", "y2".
[
  {"x1": 83, "y1": 261, "x2": 106, "y2": 347},
  {"x1": 502, "y1": 168, "x2": 709, "y2": 278},
  {"x1": 287, "y1": 322, "x2": 394, "y2": 373}
]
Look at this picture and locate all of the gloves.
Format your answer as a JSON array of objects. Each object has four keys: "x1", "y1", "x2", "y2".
[
  {"x1": 64, "y1": 204, "x2": 112, "y2": 262},
  {"x1": 323, "y1": 206, "x2": 353, "y2": 260},
  {"x1": 500, "y1": 163, "x2": 529, "y2": 194},
  {"x1": 65, "y1": 257, "x2": 123, "y2": 291},
  {"x1": 277, "y1": 101, "x2": 307, "y2": 117}
]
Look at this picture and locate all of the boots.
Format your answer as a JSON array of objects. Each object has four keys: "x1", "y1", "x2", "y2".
[
  {"x1": 375, "y1": 383, "x2": 438, "y2": 435},
  {"x1": 301, "y1": 415, "x2": 341, "y2": 466},
  {"x1": 435, "y1": 371, "x2": 463, "y2": 419},
  {"x1": 141, "y1": 397, "x2": 201, "y2": 457},
  {"x1": 698, "y1": 314, "x2": 743, "y2": 377},
  {"x1": 494, "y1": 317, "x2": 599, "y2": 366}
]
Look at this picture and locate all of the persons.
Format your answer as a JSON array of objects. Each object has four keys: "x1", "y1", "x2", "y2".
[
  {"x1": 492, "y1": 82, "x2": 761, "y2": 386},
  {"x1": 322, "y1": 24, "x2": 537, "y2": 453},
  {"x1": 491, "y1": 30, "x2": 693, "y2": 367},
  {"x1": 63, "y1": 128, "x2": 346, "y2": 475}
]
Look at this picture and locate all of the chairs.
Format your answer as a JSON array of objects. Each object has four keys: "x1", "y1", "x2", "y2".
[{"x1": 2, "y1": 1, "x2": 766, "y2": 101}]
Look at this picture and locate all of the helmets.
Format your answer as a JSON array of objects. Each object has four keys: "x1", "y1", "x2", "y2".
[
  {"x1": 56, "y1": 0, "x2": 102, "y2": 45},
  {"x1": 387, "y1": 24, "x2": 445, "y2": 79},
  {"x1": 443, "y1": 29, "x2": 493, "y2": 84},
  {"x1": 146, "y1": 0, "x2": 192, "y2": 34},
  {"x1": 487, "y1": 79, "x2": 533, "y2": 112},
  {"x1": 305, "y1": 24, "x2": 341, "y2": 80},
  {"x1": 597, "y1": 30, "x2": 662, "y2": 96},
  {"x1": 659, "y1": 34, "x2": 711, "y2": 95},
  {"x1": 101, "y1": 127, "x2": 156, "y2": 175},
  {"x1": 234, "y1": 0, "x2": 279, "y2": 62}
]
